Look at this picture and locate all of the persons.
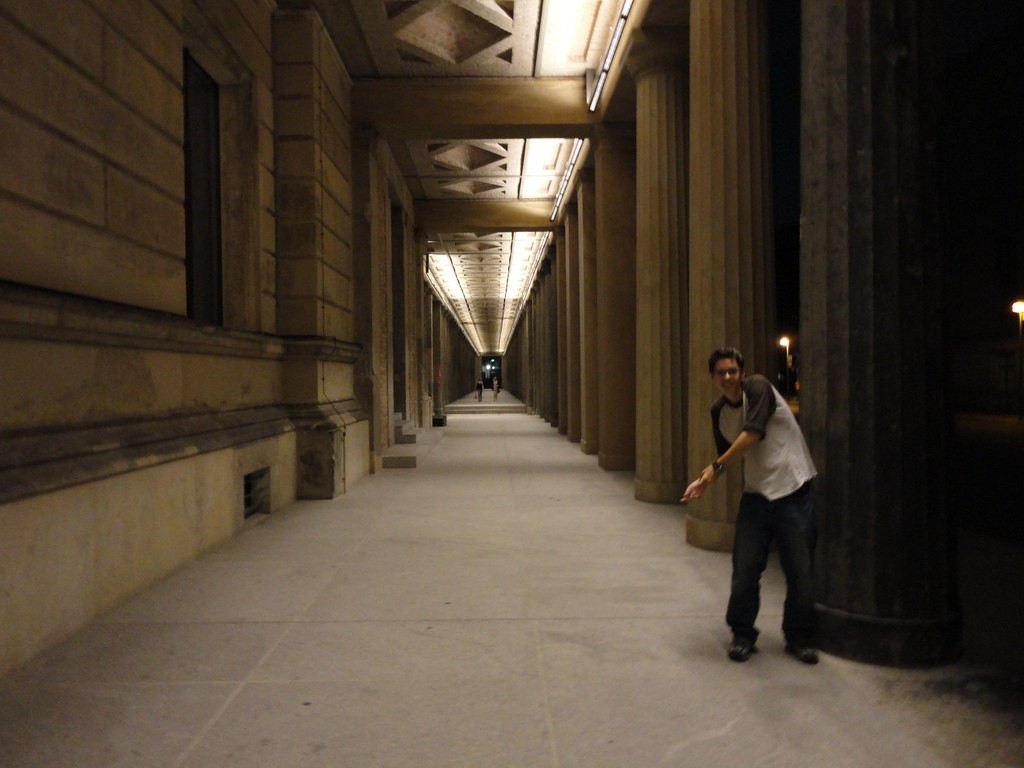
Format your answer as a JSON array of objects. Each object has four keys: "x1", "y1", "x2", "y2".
[
  {"x1": 492, "y1": 376, "x2": 499, "y2": 402},
  {"x1": 679, "y1": 346, "x2": 820, "y2": 664},
  {"x1": 474, "y1": 377, "x2": 483, "y2": 402}
]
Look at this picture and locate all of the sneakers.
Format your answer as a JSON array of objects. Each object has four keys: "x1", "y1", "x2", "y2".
[
  {"x1": 785, "y1": 635, "x2": 819, "y2": 664},
  {"x1": 728, "y1": 626, "x2": 760, "y2": 662}
]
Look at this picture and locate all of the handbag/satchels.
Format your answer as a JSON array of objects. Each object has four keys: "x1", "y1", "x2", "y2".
[
  {"x1": 497, "y1": 386, "x2": 500, "y2": 393},
  {"x1": 475, "y1": 393, "x2": 477, "y2": 399}
]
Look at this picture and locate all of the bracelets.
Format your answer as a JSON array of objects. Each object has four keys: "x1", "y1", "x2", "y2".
[{"x1": 712, "y1": 461, "x2": 727, "y2": 474}]
